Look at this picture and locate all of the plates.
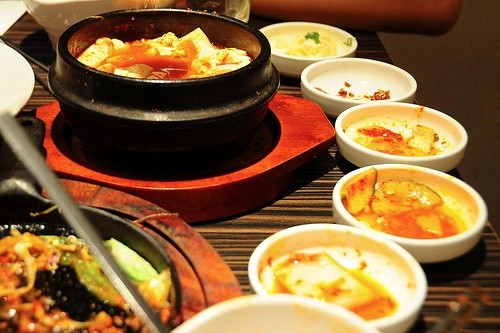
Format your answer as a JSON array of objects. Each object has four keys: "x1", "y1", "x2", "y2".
[{"x1": 0, "y1": 42, "x2": 35, "y2": 118}]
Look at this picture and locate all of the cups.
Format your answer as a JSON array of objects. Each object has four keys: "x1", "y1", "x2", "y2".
[{"x1": 170, "y1": 293, "x2": 380, "y2": 333}]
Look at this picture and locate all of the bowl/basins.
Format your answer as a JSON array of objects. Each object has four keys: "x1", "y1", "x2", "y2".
[
  {"x1": 248, "y1": 224, "x2": 427, "y2": 333},
  {"x1": 300, "y1": 57, "x2": 417, "y2": 117},
  {"x1": 48, "y1": 8, "x2": 281, "y2": 143},
  {"x1": 335, "y1": 102, "x2": 468, "y2": 172},
  {"x1": 259, "y1": 21, "x2": 358, "y2": 77},
  {"x1": 23, "y1": 0, "x2": 156, "y2": 52},
  {"x1": 0, "y1": 177, "x2": 180, "y2": 333},
  {"x1": 332, "y1": 163, "x2": 488, "y2": 263}
]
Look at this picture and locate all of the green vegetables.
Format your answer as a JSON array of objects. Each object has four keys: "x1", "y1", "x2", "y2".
[
  {"x1": 54, "y1": 233, "x2": 161, "y2": 302},
  {"x1": 343, "y1": 37, "x2": 353, "y2": 48},
  {"x1": 305, "y1": 31, "x2": 319, "y2": 44}
]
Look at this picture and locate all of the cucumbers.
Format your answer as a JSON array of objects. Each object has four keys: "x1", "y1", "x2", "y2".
[
  {"x1": 341, "y1": 168, "x2": 378, "y2": 215},
  {"x1": 371, "y1": 180, "x2": 442, "y2": 217}
]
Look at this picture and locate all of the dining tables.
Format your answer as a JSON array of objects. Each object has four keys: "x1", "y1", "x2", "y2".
[{"x1": 0, "y1": 0, "x2": 500, "y2": 333}]
